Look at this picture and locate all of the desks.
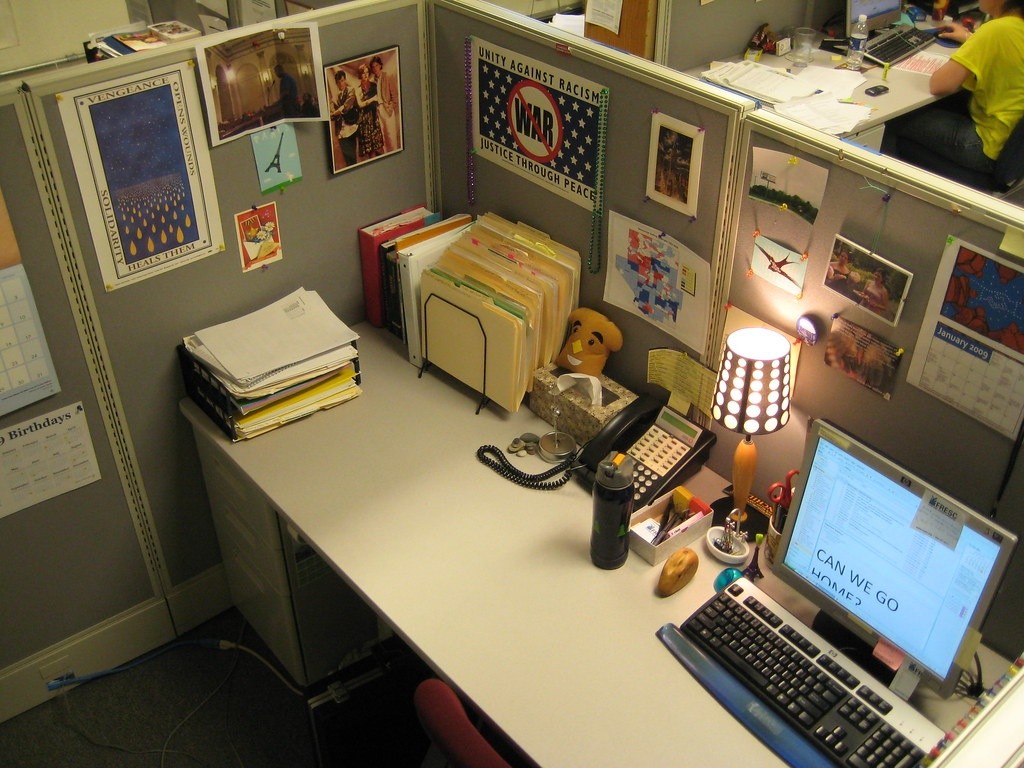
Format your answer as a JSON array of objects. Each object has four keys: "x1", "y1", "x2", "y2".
[
  {"x1": 677, "y1": 13, "x2": 984, "y2": 153},
  {"x1": 178, "y1": 317, "x2": 1016, "y2": 768}
]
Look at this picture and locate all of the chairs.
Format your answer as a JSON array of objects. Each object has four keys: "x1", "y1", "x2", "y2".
[
  {"x1": 893, "y1": 115, "x2": 1024, "y2": 195},
  {"x1": 414, "y1": 677, "x2": 513, "y2": 768}
]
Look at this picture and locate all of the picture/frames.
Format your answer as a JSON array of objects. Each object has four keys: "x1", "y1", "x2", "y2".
[
  {"x1": 646, "y1": 111, "x2": 705, "y2": 220},
  {"x1": 322, "y1": 45, "x2": 404, "y2": 175}
]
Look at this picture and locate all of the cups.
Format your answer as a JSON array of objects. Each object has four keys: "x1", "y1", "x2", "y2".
[
  {"x1": 588, "y1": 450, "x2": 636, "y2": 571},
  {"x1": 793, "y1": 27, "x2": 816, "y2": 67}
]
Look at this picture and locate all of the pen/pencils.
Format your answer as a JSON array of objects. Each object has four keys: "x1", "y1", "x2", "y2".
[
  {"x1": 774, "y1": 505, "x2": 782, "y2": 529},
  {"x1": 777, "y1": 510, "x2": 788, "y2": 534},
  {"x1": 772, "y1": 494, "x2": 778, "y2": 527},
  {"x1": 651, "y1": 512, "x2": 679, "y2": 546},
  {"x1": 655, "y1": 509, "x2": 669, "y2": 539}
]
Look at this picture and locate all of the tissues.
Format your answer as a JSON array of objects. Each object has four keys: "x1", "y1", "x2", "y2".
[{"x1": 529, "y1": 359, "x2": 638, "y2": 449}]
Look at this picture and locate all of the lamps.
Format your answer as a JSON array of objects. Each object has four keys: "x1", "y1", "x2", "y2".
[{"x1": 710, "y1": 327, "x2": 791, "y2": 540}]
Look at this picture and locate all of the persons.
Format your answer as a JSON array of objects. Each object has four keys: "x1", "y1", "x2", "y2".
[
  {"x1": 880, "y1": 0, "x2": 1024, "y2": 172},
  {"x1": 274, "y1": 65, "x2": 315, "y2": 119},
  {"x1": 330, "y1": 56, "x2": 398, "y2": 166},
  {"x1": 825, "y1": 248, "x2": 851, "y2": 294},
  {"x1": 860, "y1": 268, "x2": 889, "y2": 316}
]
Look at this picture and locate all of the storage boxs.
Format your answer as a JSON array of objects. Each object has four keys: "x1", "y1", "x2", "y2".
[
  {"x1": 744, "y1": 46, "x2": 763, "y2": 62},
  {"x1": 628, "y1": 484, "x2": 714, "y2": 566}
]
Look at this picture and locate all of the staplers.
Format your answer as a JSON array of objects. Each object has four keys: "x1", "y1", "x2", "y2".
[{"x1": 818, "y1": 39, "x2": 850, "y2": 57}]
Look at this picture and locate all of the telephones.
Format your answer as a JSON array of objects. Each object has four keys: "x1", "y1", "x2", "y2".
[{"x1": 569, "y1": 392, "x2": 717, "y2": 513}]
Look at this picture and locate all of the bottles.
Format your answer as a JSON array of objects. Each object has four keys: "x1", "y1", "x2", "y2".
[{"x1": 846, "y1": 15, "x2": 869, "y2": 70}]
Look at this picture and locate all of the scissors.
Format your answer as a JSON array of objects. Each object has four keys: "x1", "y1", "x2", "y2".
[{"x1": 767, "y1": 470, "x2": 799, "y2": 510}]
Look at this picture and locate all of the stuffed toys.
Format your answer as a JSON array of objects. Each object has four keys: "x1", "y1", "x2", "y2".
[{"x1": 556, "y1": 307, "x2": 623, "y2": 379}]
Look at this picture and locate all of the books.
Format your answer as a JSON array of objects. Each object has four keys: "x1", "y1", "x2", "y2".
[{"x1": 358, "y1": 204, "x2": 477, "y2": 369}]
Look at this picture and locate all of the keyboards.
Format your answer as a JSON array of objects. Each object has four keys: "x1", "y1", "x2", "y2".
[{"x1": 680, "y1": 578, "x2": 945, "y2": 768}]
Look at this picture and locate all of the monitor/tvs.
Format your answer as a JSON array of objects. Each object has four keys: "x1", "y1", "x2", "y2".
[{"x1": 770, "y1": 418, "x2": 1019, "y2": 698}]
